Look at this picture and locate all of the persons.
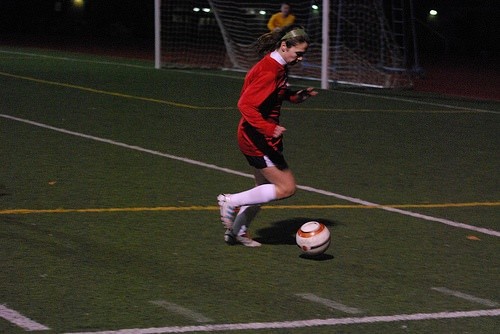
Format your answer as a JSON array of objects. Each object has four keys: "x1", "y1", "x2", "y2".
[
  {"x1": 218, "y1": 23, "x2": 319, "y2": 247},
  {"x1": 267, "y1": 2, "x2": 296, "y2": 33}
]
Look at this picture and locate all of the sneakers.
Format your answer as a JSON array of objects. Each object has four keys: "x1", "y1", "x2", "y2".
[
  {"x1": 217, "y1": 193, "x2": 237, "y2": 229},
  {"x1": 224, "y1": 230, "x2": 261, "y2": 247}
]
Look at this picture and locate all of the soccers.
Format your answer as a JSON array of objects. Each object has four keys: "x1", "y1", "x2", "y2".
[{"x1": 296, "y1": 221, "x2": 331, "y2": 256}]
